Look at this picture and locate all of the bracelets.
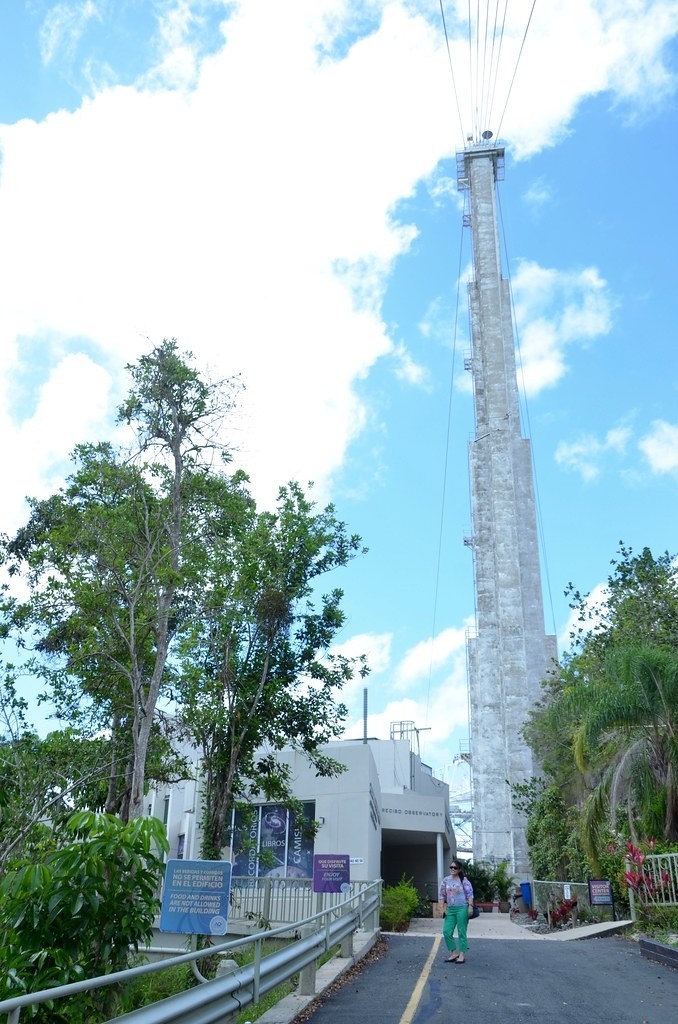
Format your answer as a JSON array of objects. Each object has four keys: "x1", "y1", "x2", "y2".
[{"x1": 469, "y1": 903, "x2": 473, "y2": 906}]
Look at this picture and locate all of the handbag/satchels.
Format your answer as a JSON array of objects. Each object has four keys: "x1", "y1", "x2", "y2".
[{"x1": 468, "y1": 903, "x2": 479, "y2": 919}]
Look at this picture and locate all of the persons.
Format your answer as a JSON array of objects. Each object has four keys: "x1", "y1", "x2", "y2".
[{"x1": 439, "y1": 860, "x2": 474, "y2": 964}]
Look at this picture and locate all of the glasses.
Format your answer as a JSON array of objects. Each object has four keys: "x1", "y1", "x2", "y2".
[{"x1": 449, "y1": 866, "x2": 458, "y2": 870}]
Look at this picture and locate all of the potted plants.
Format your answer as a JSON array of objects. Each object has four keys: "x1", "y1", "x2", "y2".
[
  {"x1": 491, "y1": 858, "x2": 522, "y2": 913},
  {"x1": 462, "y1": 858, "x2": 499, "y2": 912}
]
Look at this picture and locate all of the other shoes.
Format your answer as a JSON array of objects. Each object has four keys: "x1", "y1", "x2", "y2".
[
  {"x1": 445, "y1": 955, "x2": 459, "y2": 962},
  {"x1": 455, "y1": 958, "x2": 465, "y2": 963}
]
